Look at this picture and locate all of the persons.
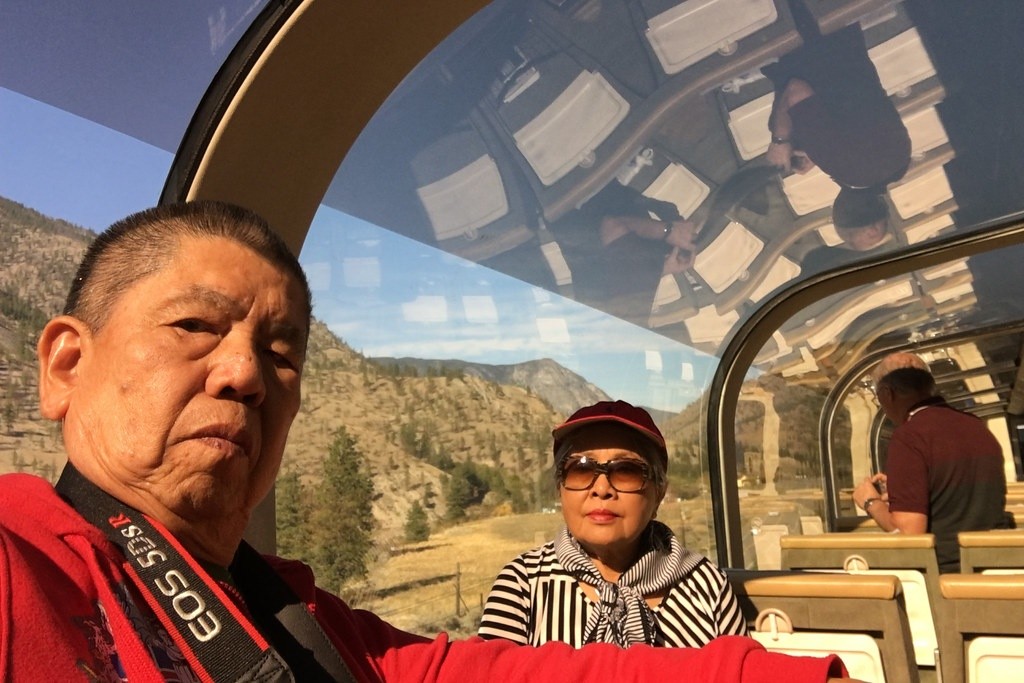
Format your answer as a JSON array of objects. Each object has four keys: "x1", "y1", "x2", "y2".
[
  {"x1": 852, "y1": 352, "x2": 1007, "y2": 574},
  {"x1": 475, "y1": 401, "x2": 752, "y2": 649},
  {"x1": 1, "y1": 201, "x2": 867, "y2": 682},
  {"x1": 479, "y1": 177, "x2": 697, "y2": 329},
  {"x1": 767, "y1": 21, "x2": 912, "y2": 249}
]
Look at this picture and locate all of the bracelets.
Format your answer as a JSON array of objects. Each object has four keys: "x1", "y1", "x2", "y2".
[
  {"x1": 662, "y1": 221, "x2": 673, "y2": 243},
  {"x1": 863, "y1": 497, "x2": 882, "y2": 513},
  {"x1": 771, "y1": 136, "x2": 793, "y2": 144}
]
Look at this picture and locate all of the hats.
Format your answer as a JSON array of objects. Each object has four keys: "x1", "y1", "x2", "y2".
[{"x1": 552, "y1": 400, "x2": 668, "y2": 475}]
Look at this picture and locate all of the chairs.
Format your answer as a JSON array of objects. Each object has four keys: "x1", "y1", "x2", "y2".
[
  {"x1": 346, "y1": 1, "x2": 978, "y2": 401},
  {"x1": 774, "y1": 479, "x2": 1024, "y2": 683},
  {"x1": 720, "y1": 565, "x2": 922, "y2": 683}
]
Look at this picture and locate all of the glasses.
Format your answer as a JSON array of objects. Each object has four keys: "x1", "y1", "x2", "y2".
[
  {"x1": 557, "y1": 455, "x2": 653, "y2": 493},
  {"x1": 871, "y1": 387, "x2": 884, "y2": 408}
]
